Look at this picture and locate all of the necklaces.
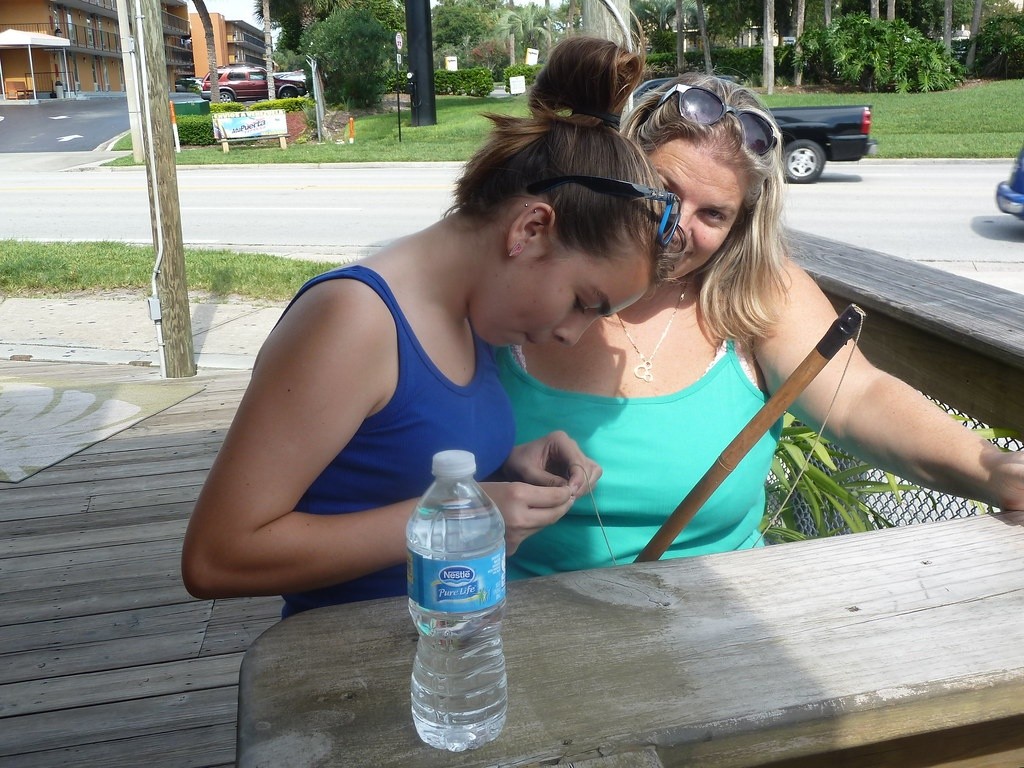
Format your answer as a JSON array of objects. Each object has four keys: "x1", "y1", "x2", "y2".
[{"x1": 617, "y1": 283, "x2": 686, "y2": 382}]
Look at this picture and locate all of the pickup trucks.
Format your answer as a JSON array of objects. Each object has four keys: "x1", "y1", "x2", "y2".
[{"x1": 631, "y1": 77, "x2": 876, "y2": 184}]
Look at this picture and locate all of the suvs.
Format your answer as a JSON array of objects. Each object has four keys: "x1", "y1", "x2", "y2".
[{"x1": 201, "y1": 62, "x2": 307, "y2": 105}]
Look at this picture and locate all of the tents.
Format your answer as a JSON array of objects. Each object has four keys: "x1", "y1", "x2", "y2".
[{"x1": 0, "y1": 29, "x2": 70, "y2": 100}]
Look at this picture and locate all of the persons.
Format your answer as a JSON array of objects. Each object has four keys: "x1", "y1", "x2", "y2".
[
  {"x1": 182, "y1": 37, "x2": 687, "y2": 622},
  {"x1": 494, "y1": 72, "x2": 1024, "y2": 582}
]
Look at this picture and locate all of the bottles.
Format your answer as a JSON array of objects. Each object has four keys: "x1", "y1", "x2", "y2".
[{"x1": 405, "y1": 450, "x2": 507, "y2": 753}]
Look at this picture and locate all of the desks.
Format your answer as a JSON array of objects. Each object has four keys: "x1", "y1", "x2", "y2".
[
  {"x1": 236, "y1": 509, "x2": 1024, "y2": 768},
  {"x1": 13, "y1": 89, "x2": 33, "y2": 100}
]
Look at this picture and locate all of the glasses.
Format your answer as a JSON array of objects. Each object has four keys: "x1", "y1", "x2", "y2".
[
  {"x1": 655, "y1": 83, "x2": 777, "y2": 155},
  {"x1": 527, "y1": 175, "x2": 682, "y2": 248}
]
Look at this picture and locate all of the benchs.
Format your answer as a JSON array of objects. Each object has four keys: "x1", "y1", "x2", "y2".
[{"x1": 5, "y1": 81, "x2": 26, "y2": 99}]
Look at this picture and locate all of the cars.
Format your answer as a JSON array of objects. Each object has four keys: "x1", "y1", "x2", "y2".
[
  {"x1": 175, "y1": 77, "x2": 205, "y2": 92},
  {"x1": 995, "y1": 136, "x2": 1024, "y2": 220}
]
